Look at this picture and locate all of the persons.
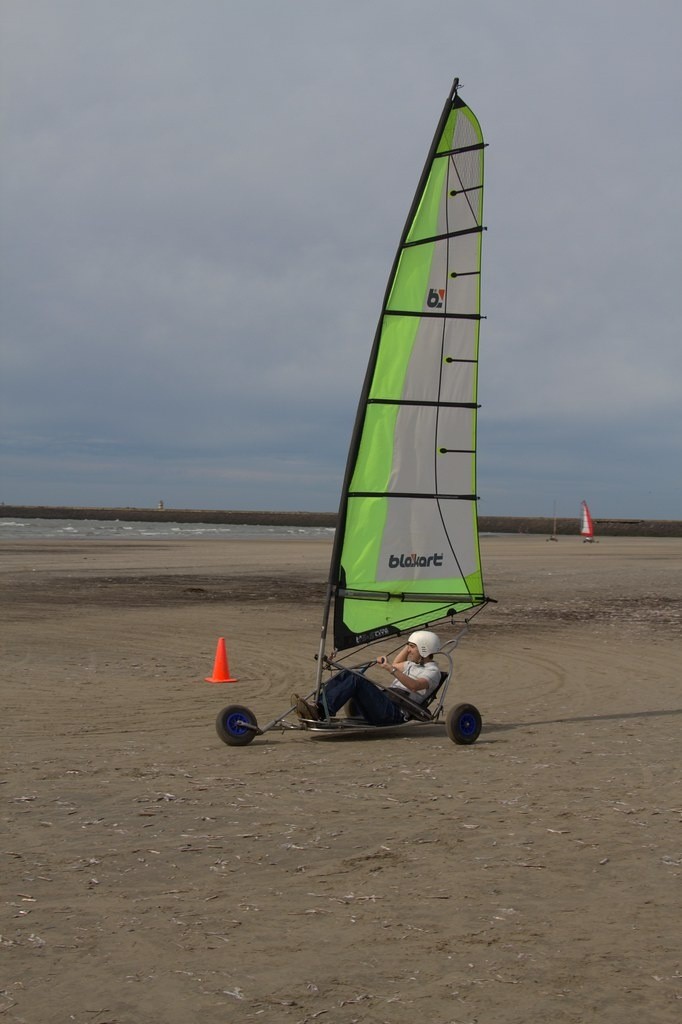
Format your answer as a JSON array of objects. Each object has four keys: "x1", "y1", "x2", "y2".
[{"x1": 290, "y1": 629, "x2": 442, "y2": 727}]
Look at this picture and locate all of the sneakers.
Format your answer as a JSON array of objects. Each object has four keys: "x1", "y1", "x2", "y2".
[
  {"x1": 298, "y1": 698, "x2": 319, "y2": 725},
  {"x1": 290, "y1": 693, "x2": 305, "y2": 721}
]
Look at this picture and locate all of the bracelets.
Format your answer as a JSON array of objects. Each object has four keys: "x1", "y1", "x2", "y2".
[{"x1": 390, "y1": 667, "x2": 397, "y2": 675}]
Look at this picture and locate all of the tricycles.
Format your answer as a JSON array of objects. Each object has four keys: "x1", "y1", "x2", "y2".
[{"x1": 214, "y1": 76, "x2": 499, "y2": 747}]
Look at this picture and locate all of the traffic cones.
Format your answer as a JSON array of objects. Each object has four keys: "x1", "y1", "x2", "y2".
[{"x1": 204, "y1": 636, "x2": 238, "y2": 683}]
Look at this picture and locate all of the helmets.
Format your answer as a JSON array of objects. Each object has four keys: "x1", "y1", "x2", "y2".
[{"x1": 408, "y1": 630, "x2": 442, "y2": 658}]
